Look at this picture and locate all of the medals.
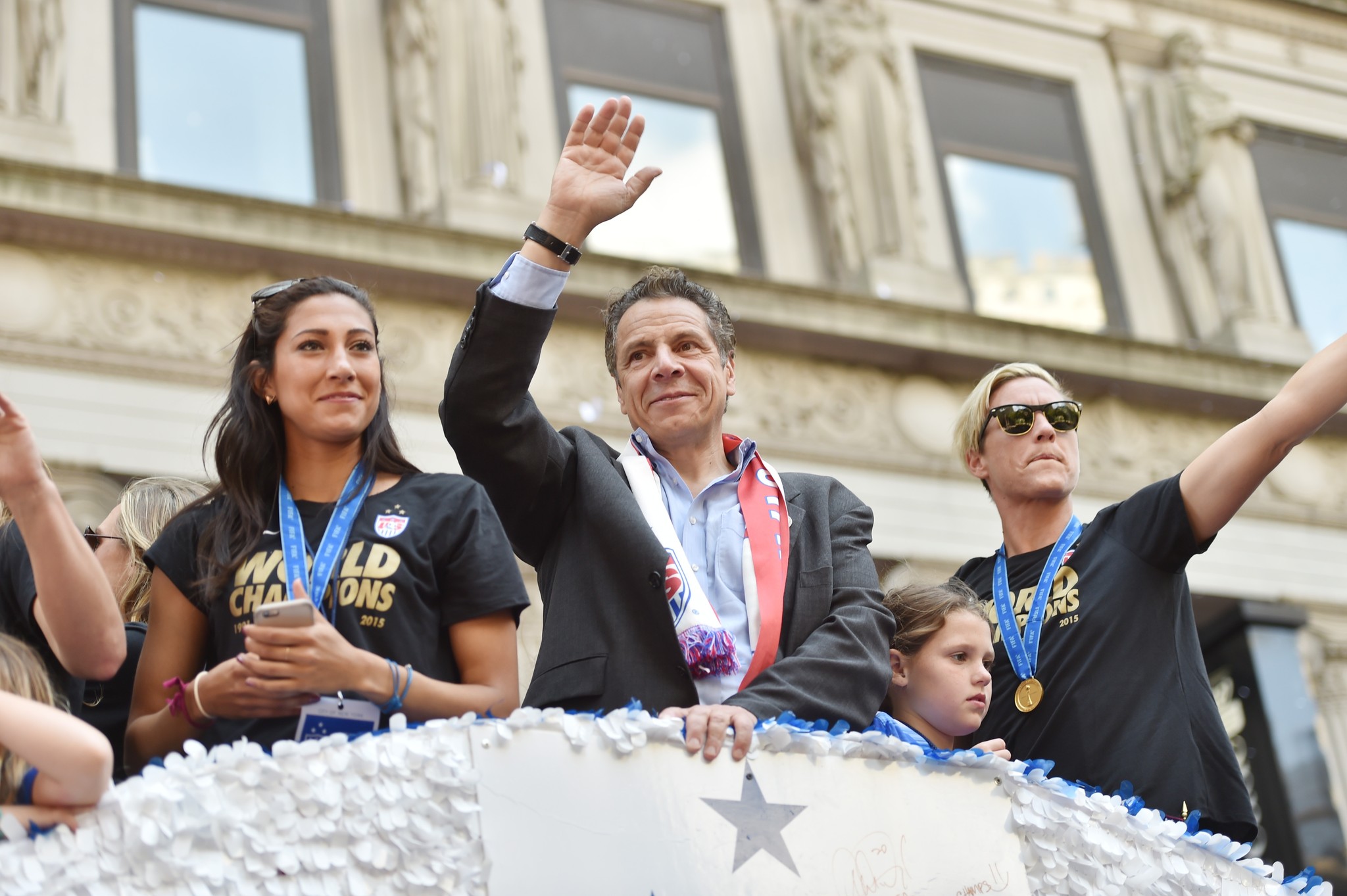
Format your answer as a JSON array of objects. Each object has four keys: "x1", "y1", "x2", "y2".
[{"x1": 1014, "y1": 676, "x2": 1043, "y2": 713}]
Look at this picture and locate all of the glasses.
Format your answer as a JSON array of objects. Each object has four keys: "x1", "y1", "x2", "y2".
[
  {"x1": 978, "y1": 399, "x2": 1082, "y2": 446},
  {"x1": 251, "y1": 275, "x2": 359, "y2": 318},
  {"x1": 83, "y1": 525, "x2": 127, "y2": 554}
]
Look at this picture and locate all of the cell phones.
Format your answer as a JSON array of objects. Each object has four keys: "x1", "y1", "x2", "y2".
[{"x1": 253, "y1": 599, "x2": 313, "y2": 628}]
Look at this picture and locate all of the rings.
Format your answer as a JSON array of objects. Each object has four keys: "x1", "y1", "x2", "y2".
[{"x1": 285, "y1": 646, "x2": 291, "y2": 660}]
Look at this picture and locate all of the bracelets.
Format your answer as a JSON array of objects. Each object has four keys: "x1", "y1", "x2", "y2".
[
  {"x1": 372, "y1": 658, "x2": 414, "y2": 714},
  {"x1": 162, "y1": 677, "x2": 215, "y2": 728},
  {"x1": 523, "y1": 220, "x2": 583, "y2": 266},
  {"x1": 194, "y1": 671, "x2": 215, "y2": 720}
]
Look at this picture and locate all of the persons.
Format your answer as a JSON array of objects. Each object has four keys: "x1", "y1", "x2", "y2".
[
  {"x1": 855, "y1": 580, "x2": 1011, "y2": 760},
  {"x1": 930, "y1": 331, "x2": 1347, "y2": 863},
  {"x1": 0, "y1": 386, "x2": 227, "y2": 846},
  {"x1": 120, "y1": 274, "x2": 532, "y2": 773}
]
